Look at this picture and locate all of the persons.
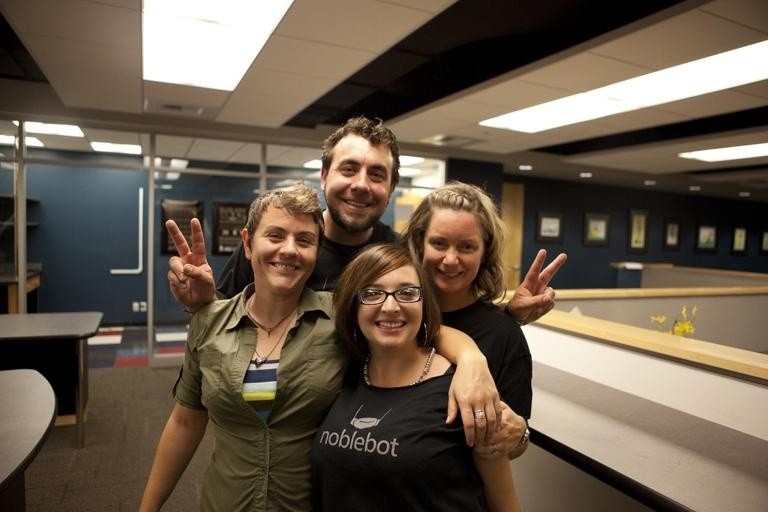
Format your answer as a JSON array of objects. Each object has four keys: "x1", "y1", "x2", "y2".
[
  {"x1": 308, "y1": 244, "x2": 524, "y2": 512},
  {"x1": 164, "y1": 116, "x2": 568, "y2": 330},
  {"x1": 400, "y1": 181, "x2": 534, "y2": 462},
  {"x1": 138, "y1": 181, "x2": 503, "y2": 511}
]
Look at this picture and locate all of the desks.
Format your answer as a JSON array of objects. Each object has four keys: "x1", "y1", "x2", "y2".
[{"x1": 0, "y1": 311, "x2": 104, "y2": 512}]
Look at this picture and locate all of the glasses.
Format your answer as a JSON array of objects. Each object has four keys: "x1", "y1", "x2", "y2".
[{"x1": 357, "y1": 287, "x2": 422, "y2": 305}]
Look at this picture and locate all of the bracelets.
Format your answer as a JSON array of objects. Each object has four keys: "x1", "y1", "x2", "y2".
[
  {"x1": 516, "y1": 418, "x2": 531, "y2": 449},
  {"x1": 502, "y1": 304, "x2": 529, "y2": 326}
]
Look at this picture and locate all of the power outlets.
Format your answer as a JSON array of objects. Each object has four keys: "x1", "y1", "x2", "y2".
[{"x1": 132, "y1": 301, "x2": 147, "y2": 313}]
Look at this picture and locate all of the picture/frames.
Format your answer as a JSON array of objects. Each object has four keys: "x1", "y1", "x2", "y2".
[
  {"x1": 160, "y1": 197, "x2": 250, "y2": 260},
  {"x1": 534, "y1": 206, "x2": 768, "y2": 254}
]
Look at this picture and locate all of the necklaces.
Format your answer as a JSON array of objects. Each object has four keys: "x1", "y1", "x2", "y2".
[
  {"x1": 249, "y1": 304, "x2": 297, "y2": 337},
  {"x1": 247, "y1": 294, "x2": 298, "y2": 367},
  {"x1": 363, "y1": 346, "x2": 435, "y2": 389}
]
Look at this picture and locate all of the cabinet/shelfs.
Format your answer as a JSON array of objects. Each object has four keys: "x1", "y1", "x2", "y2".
[{"x1": 0, "y1": 193, "x2": 45, "y2": 313}]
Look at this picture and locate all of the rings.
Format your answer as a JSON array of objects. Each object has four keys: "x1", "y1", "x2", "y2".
[
  {"x1": 489, "y1": 443, "x2": 497, "y2": 455},
  {"x1": 473, "y1": 410, "x2": 486, "y2": 419}
]
